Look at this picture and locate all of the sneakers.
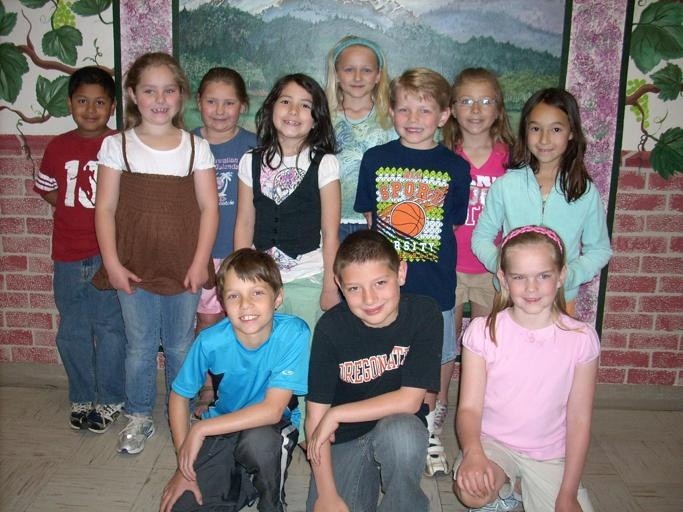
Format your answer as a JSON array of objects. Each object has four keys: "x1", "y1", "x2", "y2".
[
  {"x1": 115, "y1": 410, "x2": 155, "y2": 455},
  {"x1": 423, "y1": 431, "x2": 448, "y2": 478},
  {"x1": 69, "y1": 398, "x2": 90, "y2": 428},
  {"x1": 87, "y1": 402, "x2": 122, "y2": 434}
]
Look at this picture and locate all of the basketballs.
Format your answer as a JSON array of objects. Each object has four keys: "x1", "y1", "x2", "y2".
[{"x1": 391, "y1": 200, "x2": 426, "y2": 240}]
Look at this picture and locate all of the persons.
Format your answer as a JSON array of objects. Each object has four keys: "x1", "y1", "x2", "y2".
[
  {"x1": 302, "y1": 228, "x2": 444, "y2": 512},
  {"x1": 160, "y1": 246, "x2": 313, "y2": 512},
  {"x1": 94, "y1": 51, "x2": 220, "y2": 457},
  {"x1": 470, "y1": 87, "x2": 614, "y2": 320},
  {"x1": 231, "y1": 71, "x2": 343, "y2": 312},
  {"x1": 188, "y1": 66, "x2": 270, "y2": 419},
  {"x1": 452, "y1": 224, "x2": 602, "y2": 512},
  {"x1": 326, "y1": 33, "x2": 398, "y2": 243},
  {"x1": 432, "y1": 66, "x2": 519, "y2": 435},
  {"x1": 353, "y1": 66, "x2": 471, "y2": 478},
  {"x1": 32, "y1": 64, "x2": 137, "y2": 434}
]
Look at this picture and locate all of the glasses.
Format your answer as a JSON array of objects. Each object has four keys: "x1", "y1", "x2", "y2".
[{"x1": 452, "y1": 97, "x2": 499, "y2": 109}]
